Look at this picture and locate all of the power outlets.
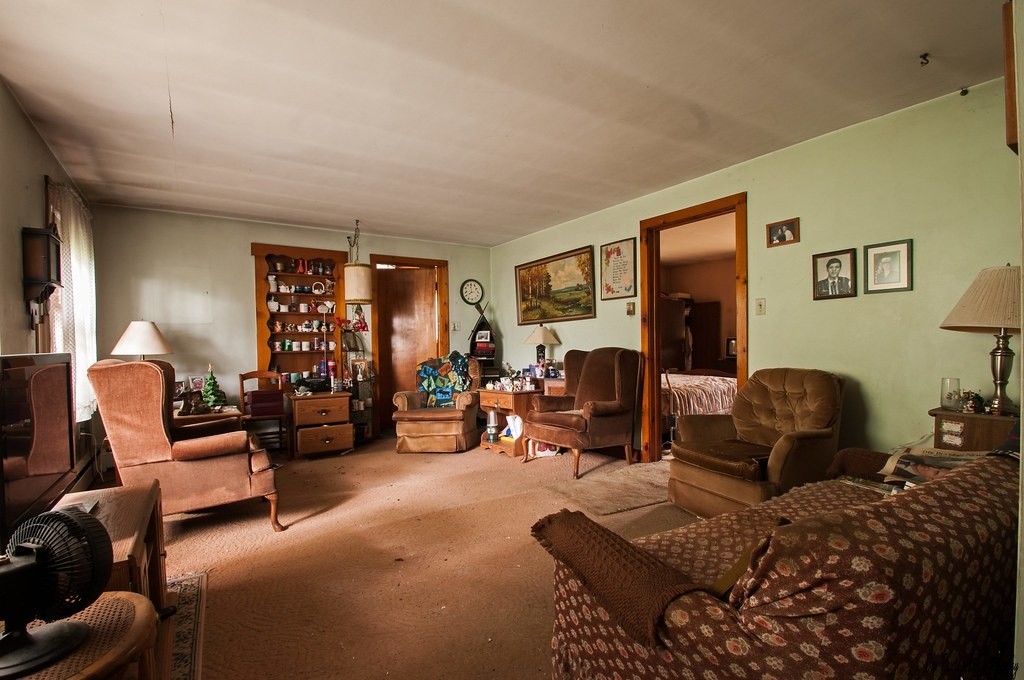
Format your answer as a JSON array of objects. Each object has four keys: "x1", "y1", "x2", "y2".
[{"x1": 77, "y1": 422, "x2": 87, "y2": 440}]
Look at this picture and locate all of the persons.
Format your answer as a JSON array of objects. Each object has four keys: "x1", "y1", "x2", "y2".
[
  {"x1": 877, "y1": 254, "x2": 898, "y2": 282},
  {"x1": 892, "y1": 454, "x2": 952, "y2": 482},
  {"x1": 175, "y1": 384, "x2": 183, "y2": 393},
  {"x1": 192, "y1": 377, "x2": 203, "y2": 390},
  {"x1": 354, "y1": 363, "x2": 365, "y2": 378},
  {"x1": 773, "y1": 226, "x2": 793, "y2": 244},
  {"x1": 817, "y1": 258, "x2": 850, "y2": 296}
]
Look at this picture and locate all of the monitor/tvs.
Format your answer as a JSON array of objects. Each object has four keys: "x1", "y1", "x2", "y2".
[{"x1": 725, "y1": 337, "x2": 737, "y2": 358}]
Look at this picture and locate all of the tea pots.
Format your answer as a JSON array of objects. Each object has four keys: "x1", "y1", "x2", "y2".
[
  {"x1": 317, "y1": 299, "x2": 335, "y2": 313},
  {"x1": 279, "y1": 281, "x2": 325, "y2": 294}
]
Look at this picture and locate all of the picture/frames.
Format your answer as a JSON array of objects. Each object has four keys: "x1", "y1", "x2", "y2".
[
  {"x1": 188, "y1": 375, "x2": 205, "y2": 392},
  {"x1": 600, "y1": 237, "x2": 637, "y2": 301},
  {"x1": 863, "y1": 239, "x2": 912, "y2": 294},
  {"x1": 174, "y1": 382, "x2": 185, "y2": 397},
  {"x1": 766, "y1": 217, "x2": 801, "y2": 248},
  {"x1": 343, "y1": 330, "x2": 359, "y2": 352},
  {"x1": 351, "y1": 359, "x2": 369, "y2": 381},
  {"x1": 812, "y1": 248, "x2": 857, "y2": 302},
  {"x1": 514, "y1": 245, "x2": 596, "y2": 326}
]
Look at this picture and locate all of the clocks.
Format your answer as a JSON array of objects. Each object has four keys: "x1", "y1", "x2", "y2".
[{"x1": 460, "y1": 278, "x2": 484, "y2": 305}]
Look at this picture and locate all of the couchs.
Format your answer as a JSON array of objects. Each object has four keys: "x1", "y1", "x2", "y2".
[
  {"x1": 668, "y1": 367, "x2": 846, "y2": 519},
  {"x1": 392, "y1": 350, "x2": 482, "y2": 453},
  {"x1": 531, "y1": 446, "x2": 1020, "y2": 680}
]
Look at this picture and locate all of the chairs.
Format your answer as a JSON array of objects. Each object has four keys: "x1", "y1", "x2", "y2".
[
  {"x1": 239, "y1": 371, "x2": 292, "y2": 459},
  {"x1": 27, "y1": 363, "x2": 72, "y2": 474},
  {"x1": 87, "y1": 359, "x2": 285, "y2": 532},
  {"x1": 127, "y1": 478, "x2": 178, "y2": 680},
  {"x1": 521, "y1": 347, "x2": 644, "y2": 479}
]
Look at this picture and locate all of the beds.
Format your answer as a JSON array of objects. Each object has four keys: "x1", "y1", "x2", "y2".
[{"x1": 660, "y1": 368, "x2": 738, "y2": 434}]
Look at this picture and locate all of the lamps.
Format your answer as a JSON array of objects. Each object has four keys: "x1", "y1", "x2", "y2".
[
  {"x1": 525, "y1": 322, "x2": 559, "y2": 363},
  {"x1": 939, "y1": 262, "x2": 1020, "y2": 415},
  {"x1": 344, "y1": 220, "x2": 373, "y2": 304},
  {"x1": 110, "y1": 318, "x2": 174, "y2": 361}
]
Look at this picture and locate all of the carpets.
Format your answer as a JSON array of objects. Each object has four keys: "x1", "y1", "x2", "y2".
[
  {"x1": 523, "y1": 451, "x2": 670, "y2": 517},
  {"x1": 167, "y1": 567, "x2": 216, "y2": 680}
]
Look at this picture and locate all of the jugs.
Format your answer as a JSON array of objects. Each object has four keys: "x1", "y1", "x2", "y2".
[{"x1": 267, "y1": 275, "x2": 277, "y2": 292}]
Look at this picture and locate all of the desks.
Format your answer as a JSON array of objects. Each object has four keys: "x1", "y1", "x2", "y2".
[
  {"x1": 52, "y1": 482, "x2": 152, "y2": 592},
  {"x1": 928, "y1": 407, "x2": 1020, "y2": 450},
  {"x1": 0, "y1": 592, "x2": 159, "y2": 680},
  {"x1": 530, "y1": 377, "x2": 566, "y2": 396}
]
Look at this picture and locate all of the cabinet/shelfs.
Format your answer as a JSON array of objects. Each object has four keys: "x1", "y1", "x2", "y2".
[
  {"x1": 250, "y1": 242, "x2": 347, "y2": 391},
  {"x1": 660, "y1": 299, "x2": 697, "y2": 370},
  {"x1": 287, "y1": 391, "x2": 353, "y2": 461},
  {"x1": 476, "y1": 389, "x2": 544, "y2": 459},
  {"x1": 466, "y1": 352, "x2": 502, "y2": 419},
  {"x1": 340, "y1": 331, "x2": 370, "y2": 382}
]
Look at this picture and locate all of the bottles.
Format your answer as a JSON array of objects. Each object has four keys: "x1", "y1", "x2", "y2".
[
  {"x1": 288, "y1": 256, "x2": 332, "y2": 275},
  {"x1": 290, "y1": 295, "x2": 297, "y2": 312},
  {"x1": 357, "y1": 369, "x2": 364, "y2": 381},
  {"x1": 334, "y1": 379, "x2": 338, "y2": 386},
  {"x1": 312, "y1": 354, "x2": 336, "y2": 378}
]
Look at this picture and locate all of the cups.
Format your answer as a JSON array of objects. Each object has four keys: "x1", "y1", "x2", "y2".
[
  {"x1": 274, "y1": 337, "x2": 336, "y2": 350},
  {"x1": 547, "y1": 369, "x2": 560, "y2": 378},
  {"x1": 267, "y1": 302, "x2": 289, "y2": 312},
  {"x1": 271, "y1": 370, "x2": 311, "y2": 383},
  {"x1": 300, "y1": 303, "x2": 312, "y2": 313},
  {"x1": 351, "y1": 399, "x2": 365, "y2": 411},
  {"x1": 366, "y1": 398, "x2": 372, "y2": 407}
]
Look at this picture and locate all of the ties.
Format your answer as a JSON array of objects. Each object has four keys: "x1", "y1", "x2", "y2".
[{"x1": 831, "y1": 281, "x2": 836, "y2": 296}]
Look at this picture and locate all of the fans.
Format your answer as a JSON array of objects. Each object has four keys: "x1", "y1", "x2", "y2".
[{"x1": 0, "y1": 507, "x2": 114, "y2": 680}]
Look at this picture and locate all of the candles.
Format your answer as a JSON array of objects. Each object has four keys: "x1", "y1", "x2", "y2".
[{"x1": 331, "y1": 376, "x2": 334, "y2": 387}]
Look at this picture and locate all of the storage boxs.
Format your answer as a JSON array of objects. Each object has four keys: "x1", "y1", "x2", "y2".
[{"x1": 247, "y1": 389, "x2": 284, "y2": 416}]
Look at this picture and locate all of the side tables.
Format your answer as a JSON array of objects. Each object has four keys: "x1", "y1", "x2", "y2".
[{"x1": 174, "y1": 406, "x2": 241, "y2": 427}]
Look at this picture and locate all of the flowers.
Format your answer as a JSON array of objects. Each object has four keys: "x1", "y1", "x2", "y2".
[
  {"x1": 336, "y1": 316, "x2": 351, "y2": 331},
  {"x1": 947, "y1": 388, "x2": 981, "y2": 412}
]
